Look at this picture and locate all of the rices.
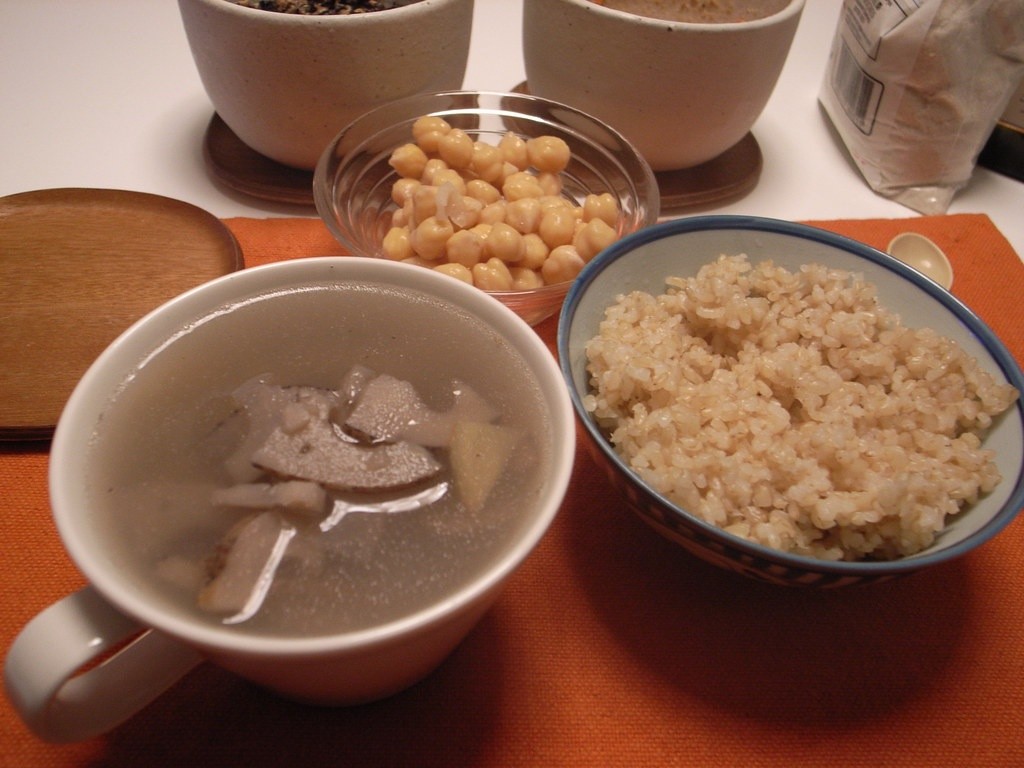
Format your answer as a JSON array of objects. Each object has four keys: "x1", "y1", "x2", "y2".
[{"x1": 584, "y1": 253, "x2": 1023, "y2": 559}]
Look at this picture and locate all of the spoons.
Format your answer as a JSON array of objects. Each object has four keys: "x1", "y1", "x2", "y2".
[{"x1": 887, "y1": 231, "x2": 954, "y2": 292}]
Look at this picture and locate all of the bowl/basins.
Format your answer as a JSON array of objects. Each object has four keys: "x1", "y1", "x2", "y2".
[
  {"x1": 313, "y1": 90, "x2": 660, "y2": 329},
  {"x1": 175, "y1": 0, "x2": 475, "y2": 174},
  {"x1": 556, "y1": 215, "x2": 1024, "y2": 591},
  {"x1": 520, "y1": 0, "x2": 807, "y2": 172},
  {"x1": 2, "y1": 256, "x2": 578, "y2": 746}
]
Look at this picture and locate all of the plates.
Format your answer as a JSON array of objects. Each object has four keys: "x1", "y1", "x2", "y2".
[
  {"x1": 191, "y1": 96, "x2": 481, "y2": 208},
  {"x1": 496, "y1": 78, "x2": 768, "y2": 213},
  {"x1": 1, "y1": 185, "x2": 248, "y2": 434}
]
